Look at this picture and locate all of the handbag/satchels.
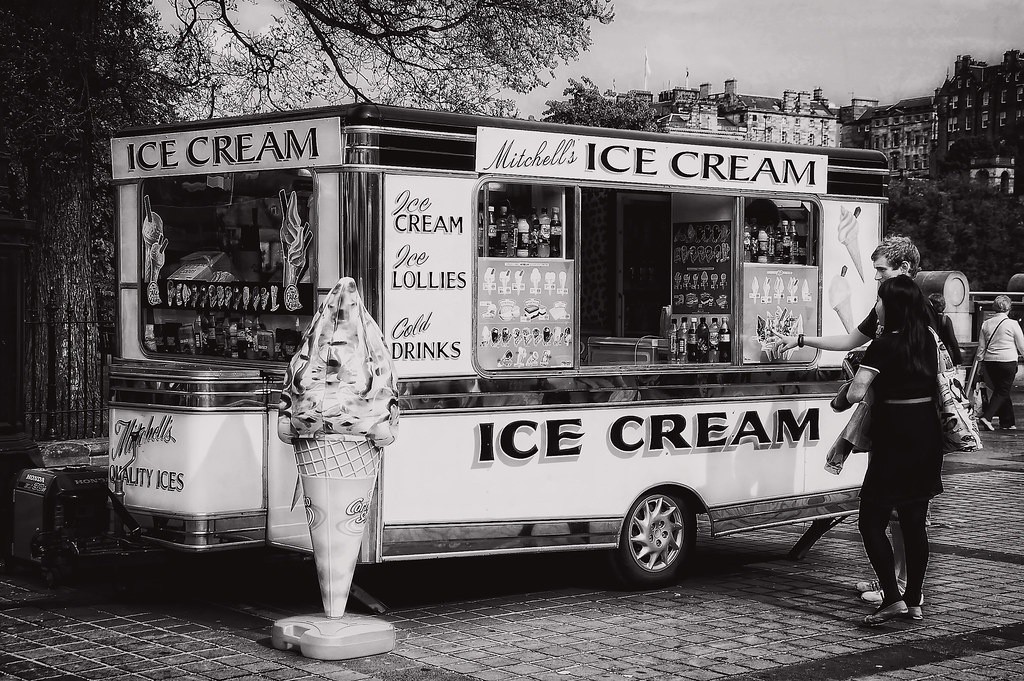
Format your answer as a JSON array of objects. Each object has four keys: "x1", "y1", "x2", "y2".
[
  {"x1": 928, "y1": 327, "x2": 983, "y2": 456},
  {"x1": 974, "y1": 381, "x2": 988, "y2": 418}
]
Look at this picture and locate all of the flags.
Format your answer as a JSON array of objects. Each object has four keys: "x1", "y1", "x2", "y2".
[{"x1": 644, "y1": 50, "x2": 649, "y2": 76}]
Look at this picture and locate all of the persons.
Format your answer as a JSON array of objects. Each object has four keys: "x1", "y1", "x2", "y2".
[
  {"x1": 845, "y1": 275, "x2": 946, "y2": 626},
  {"x1": 975, "y1": 295, "x2": 1024, "y2": 431},
  {"x1": 744, "y1": 199, "x2": 779, "y2": 231},
  {"x1": 772, "y1": 236, "x2": 960, "y2": 607}
]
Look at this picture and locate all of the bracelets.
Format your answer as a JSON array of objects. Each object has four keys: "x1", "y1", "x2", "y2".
[{"x1": 798, "y1": 334, "x2": 804, "y2": 347}]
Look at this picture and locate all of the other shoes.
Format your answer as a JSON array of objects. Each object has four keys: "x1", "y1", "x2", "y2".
[
  {"x1": 980, "y1": 418, "x2": 994, "y2": 431},
  {"x1": 862, "y1": 600, "x2": 909, "y2": 626},
  {"x1": 861, "y1": 584, "x2": 924, "y2": 605},
  {"x1": 1003, "y1": 425, "x2": 1017, "y2": 430},
  {"x1": 855, "y1": 580, "x2": 886, "y2": 593},
  {"x1": 906, "y1": 606, "x2": 924, "y2": 621}
]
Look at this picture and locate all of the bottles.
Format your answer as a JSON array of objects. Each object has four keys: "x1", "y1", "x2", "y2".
[
  {"x1": 720, "y1": 317, "x2": 731, "y2": 362},
  {"x1": 488, "y1": 206, "x2": 561, "y2": 258},
  {"x1": 667, "y1": 319, "x2": 678, "y2": 364},
  {"x1": 744, "y1": 219, "x2": 799, "y2": 266},
  {"x1": 697, "y1": 317, "x2": 709, "y2": 363},
  {"x1": 688, "y1": 318, "x2": 698, "y2": 363},
  {"x1": 708, "y1": 318, "x2": 720, "y2": 362},
  {"x1": 678, "y1": 317, "x2": 689, "y2": 364}
]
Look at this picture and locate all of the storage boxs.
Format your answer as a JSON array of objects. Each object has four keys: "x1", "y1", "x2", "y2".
[
  {"x1": 167, "y1": 251, "x2": 234, "y2": 280},
  {"x1": 589, "y1": 337, "x2": 668, "y2": 364},
  {"x1": 11, "y1": 466, "x2": 101, "y2": 562}
]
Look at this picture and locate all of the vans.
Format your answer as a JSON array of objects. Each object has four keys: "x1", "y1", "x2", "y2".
[{"x1": 105, "y1": 102, "x2": 888, "y2": 585}]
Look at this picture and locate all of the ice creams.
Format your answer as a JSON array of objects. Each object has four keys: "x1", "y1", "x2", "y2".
[
  {"x1": 277, "y1": 276, "x2": 399, "y2": 620},
  {"x1": 838, "y1": 205, "x2": 865, "y2": 283},
  {"x1": 279, "y1": 190, "x2": 314, "y2": 290},
  {"x1": 673, "y1": 271, "x2": 727, "y2": 290},
  {"x1": 829, "y1": 265, "x2": 855, "y2": 333},
  {"x1": 484, "y1": 267, "x2": 566, "y2": 296},
  {"x1": 756, "y1": 306, "x2": 804, "y2": 361},
  {"x1": 141, "y1": 194, "x2": 168, "y2": 283}
]
